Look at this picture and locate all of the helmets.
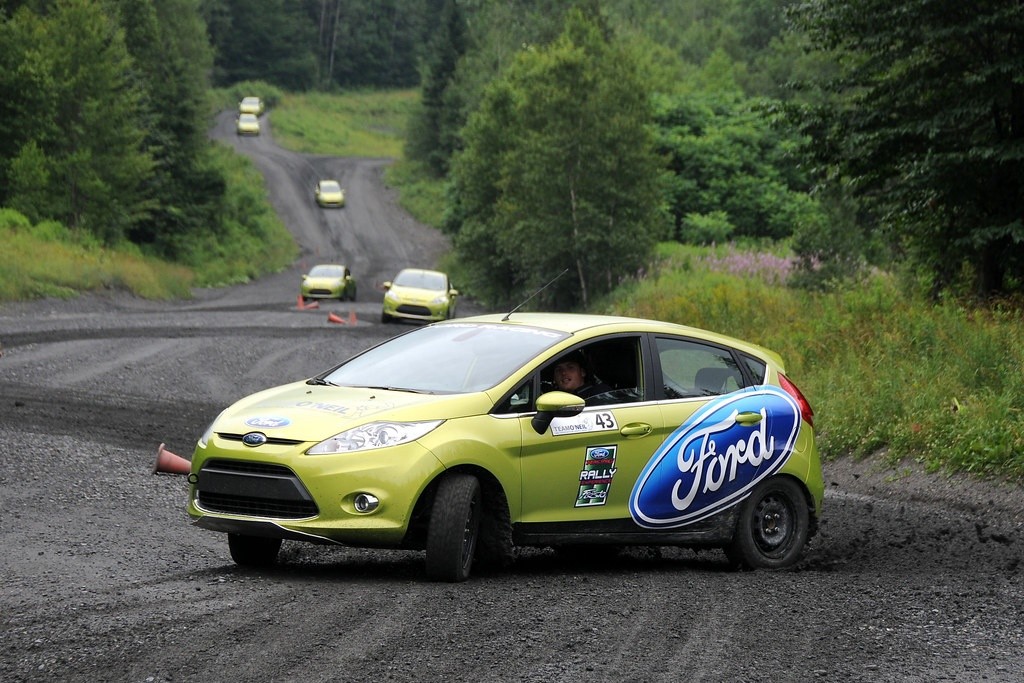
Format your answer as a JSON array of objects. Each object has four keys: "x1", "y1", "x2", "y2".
[{"x1": 551, "y1": 350, "x2": 586, "y2": 368}]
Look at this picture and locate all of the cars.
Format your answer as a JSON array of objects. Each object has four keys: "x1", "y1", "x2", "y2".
[
  {"x1": 301, "y1": 263, "x2": 358, "y2": 302},
  {"x1": 239, "y1": 96, "x2": 266, "y2": 117},
  {"x1": 235, "y1": 113, "x2": 262, "y2": 135},
  {"x1": 379, "y1": 268, "x2": 459, "y2": 329},
  {"x1": 313, "y1": 179, "x2": 347, "y2": 209},
  {"x1": 181, "y1": 311, "x2": 828, "y2": 581}
]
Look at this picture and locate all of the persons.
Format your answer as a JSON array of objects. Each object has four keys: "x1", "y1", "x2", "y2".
[{"x1": 553, "y1": 350, "x2": 596, "y2": 398}]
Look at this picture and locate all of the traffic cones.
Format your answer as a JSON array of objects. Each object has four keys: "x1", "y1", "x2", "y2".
[
  {"x1": 151, "y1": 441, "x2": 192, "y2": 477},
  {"x1": 326, "y1": 311, "x2": 344, "y2": 324},
  {"x1": 306, "y1": 300, "x2": 321, "y2": 308},
  {"x1": 347, "y1": 312, "x2": 357, "y2": 325},
  {"x1": 296, "y1": 295, "x2": 305, "y2": 312}
]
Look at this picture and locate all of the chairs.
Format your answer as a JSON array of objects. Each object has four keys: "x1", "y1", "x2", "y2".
[
  {"x1": 692, "y1": 367, "x2": 733, "y2": 395},
  {"x1": 584, "y1": 341, "x2": 638, "y2": 400}
]
